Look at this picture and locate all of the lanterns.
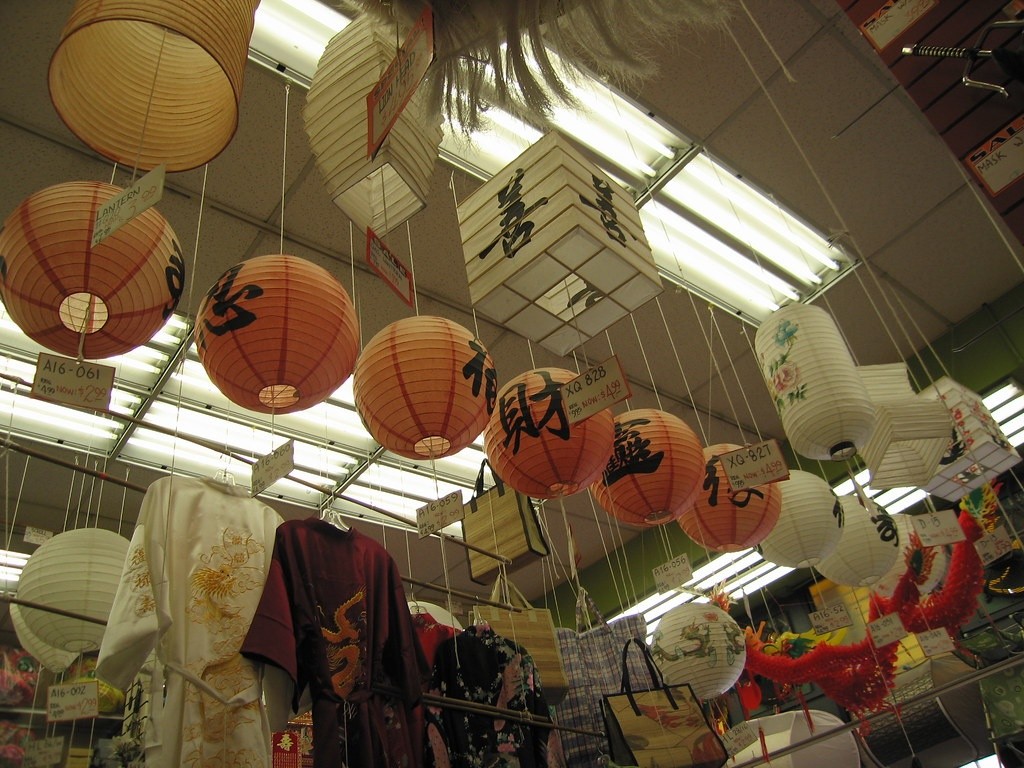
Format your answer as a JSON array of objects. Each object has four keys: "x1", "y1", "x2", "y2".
[
  {"x1": 354, "y1": 315, "x2": 498, "y2": 460},
  {"x1": 47, "y1": 1, "x2": 263, "y2": 173},
  {"x1": 16, "y1": 529, "x2": 131, "y2": 653},
  {"x1": 457, "y1": 133, "x2": 665, "y2": 359},
  {"x1": 754, "y1": 304, "x2": 875, "y2": 461},
  {"x1": 195, "y1": 255, "x2": 360, "y2": 413},
  {"x1": 854, "y1": 361, "x2": 952, "y2": 489},
  {"x1": 652, "y1": 603, "x2": 747, "y2": 701},
  {"x1": 750, "y1": 469, "x2": 844, "y2": 568},
  {"x1": 915, "y1": 375, "x2": 1022, "y2": 501},
  {"x1": 813, "y1": 494, "x2": 899, "y2": 586},
  {"x1": 677, "y1": 443, "x2": 782, "y2": 552},
  {"x1": 0, "y1": 180, "x2": 184, "y2": 360},
  {"x1": 301, "y1": 11, "x2": 445, "y2": 244},
  {"x1": 484, "y1": 367, "x2": 614, "y2": 499},
  {"x1": 875, "y1": 513, "x2": 945, "y2": 599},
  {"x1": 9, "y1": 593, "x2": 80, "y2": 673},
  {"x1": 589, "y1": 408, "x2": 705, "y2": 526}
]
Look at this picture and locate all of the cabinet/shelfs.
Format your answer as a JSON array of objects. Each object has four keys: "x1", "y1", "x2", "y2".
[{"x1": 0, "y1": 593, "x2": 127, "y2": 767}]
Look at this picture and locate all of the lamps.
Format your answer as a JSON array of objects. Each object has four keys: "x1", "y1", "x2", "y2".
[
  {"x1": 17, "y1": 529, "x2": 131, "y2": 654},
  {"x1": 8, "y1": 601, "x2": 79, "y2": 673},
  {"x1": 0, "y1": 0, "x2": 1023, "y2": 599},
  {"x1": 650, "y1": 603, "x2": 746, "y2": 699}
]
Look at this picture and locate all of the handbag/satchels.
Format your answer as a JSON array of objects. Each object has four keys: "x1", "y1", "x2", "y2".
[
  {"x1": 542, "y1": 584, "x2": 655, "y2": 762},
  {"x1": 472, "y1": 576, "x2": 569, "y2": 699},
  {"x1": 599, "y1": 635, "x2": 727, "y2": 767}
]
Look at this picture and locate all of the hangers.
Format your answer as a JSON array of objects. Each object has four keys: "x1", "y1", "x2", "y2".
[
  {"x1": 472, "y1": 595, "x2": 489, "y2": 627},
  {"x1": 409, "y1": 583, "x2": 425, "y2": 613}
]
